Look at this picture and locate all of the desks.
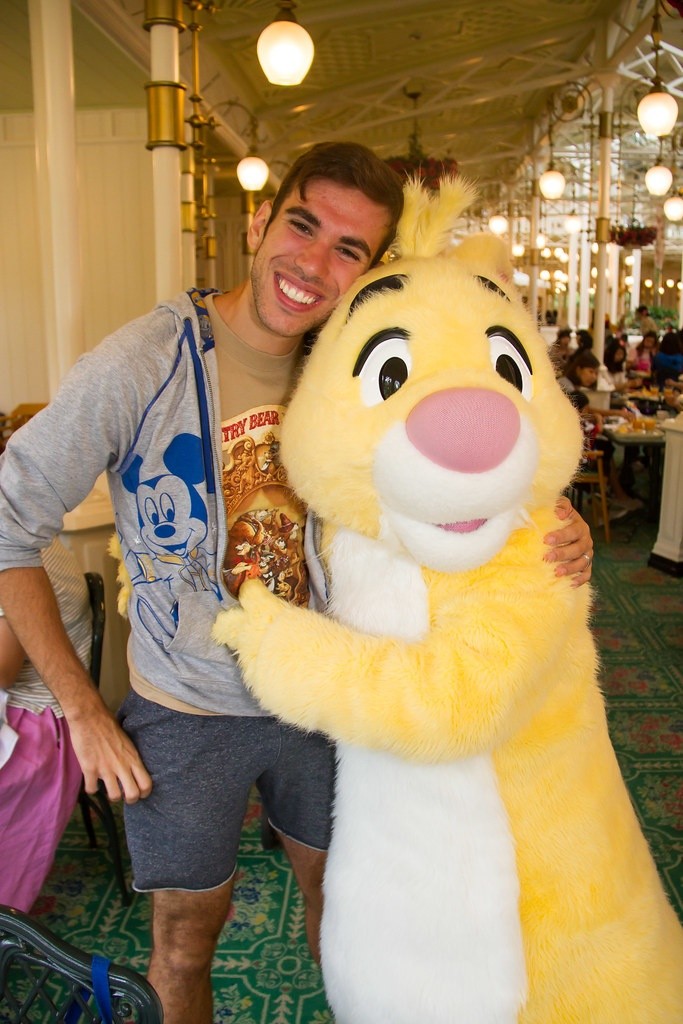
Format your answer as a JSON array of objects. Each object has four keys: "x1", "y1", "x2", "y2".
[{"x1": 598, "y1": 411, "x2": 675, "y2": 524}]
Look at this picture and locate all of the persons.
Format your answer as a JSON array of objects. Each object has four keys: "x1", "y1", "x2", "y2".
[
  {"x1": 0, "y1": 135, "x2": 595, "y2": 1024},
  {"x1": 1, "y1": 410, "x2": 94, "y2": 915},
  {"x1": 545, "y1": 304, "x2": 683, "y2": 528}
]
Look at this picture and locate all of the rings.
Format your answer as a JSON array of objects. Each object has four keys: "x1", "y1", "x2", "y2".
[{"x1": 582, "y1": 553, "x2": 591, "y2": 570}]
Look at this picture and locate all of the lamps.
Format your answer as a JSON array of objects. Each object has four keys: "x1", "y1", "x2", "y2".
[
  {"x1": 201, "y1": 99, "x2": 271, "y2": 192},
  {"x1": 254, "y1": 0, "x2": 315, "y2": 89},
  {"x1": 484, "y1": 0, "x2": 683, "y2": 237}
]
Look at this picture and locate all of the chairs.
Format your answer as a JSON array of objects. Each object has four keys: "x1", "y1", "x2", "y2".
[
  {"x1": 561, "y1": 449, "x2": 614, "y2": 546},
  {"x1": 77, "y1": 570, "x2": 135, "y2": 903}
]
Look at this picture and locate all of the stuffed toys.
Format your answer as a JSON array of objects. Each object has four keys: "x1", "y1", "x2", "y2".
[{"x1": 210, "y1": 170, "x2": 682, "y2": 1024}]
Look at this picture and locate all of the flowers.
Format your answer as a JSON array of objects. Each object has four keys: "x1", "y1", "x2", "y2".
[
  {"x1": 607, "y1": 227, "x2": 657, "y2": 246},
  {"x1": 385, "y1": 150, "x2": 461, "y2": 193}
]
